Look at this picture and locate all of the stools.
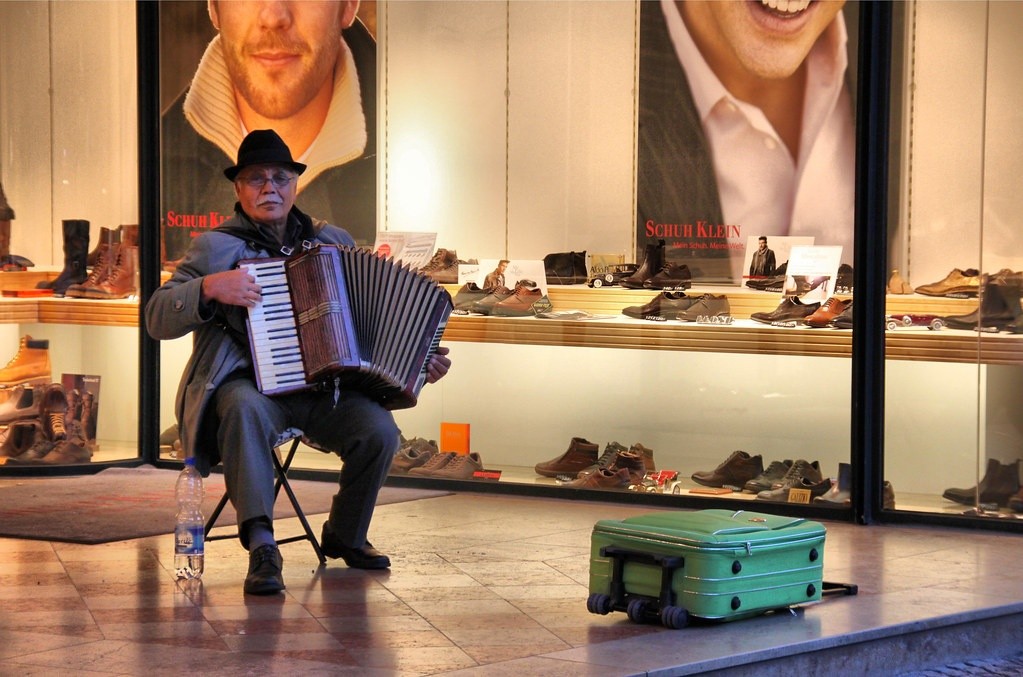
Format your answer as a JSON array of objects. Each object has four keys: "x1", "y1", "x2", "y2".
[{"x1": 200, "y1": 430, "x2": 328, "y2": 566}]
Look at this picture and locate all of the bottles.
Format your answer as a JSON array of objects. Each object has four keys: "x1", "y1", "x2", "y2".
[{"x1": 173, "y1": 457, "x2": 205, "y2": 580}]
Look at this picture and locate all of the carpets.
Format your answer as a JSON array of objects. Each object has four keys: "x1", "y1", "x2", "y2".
[{"x1": 1, "y1": 450, "x2": 459, "y2": 544}]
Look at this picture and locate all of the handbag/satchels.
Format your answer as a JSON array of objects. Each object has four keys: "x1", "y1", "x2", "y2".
[{"x1": 545, "y1": 251, "x2": 588, "y2": 285}]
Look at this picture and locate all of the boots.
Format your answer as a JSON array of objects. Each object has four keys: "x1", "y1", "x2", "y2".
[
  {"x1": 618, "y1": 240, "x2": 666, "y2": 289},
  {"x1": 944, "y1": 278, "x2": 1023, "y2": 336},
  {"x1": 0, "y1": 334, "x2": 94, "y2": 467},
  {"x1": 36, "y1": 219, "x2": 140, "y2": 298},
  {"x1": 415, "y1": 248, "x2": 458, "y2": 283}
]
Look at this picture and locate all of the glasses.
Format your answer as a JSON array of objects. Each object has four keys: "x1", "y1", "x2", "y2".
[{"x1": 236, "y1": 173, "x2": 298, "y2": 187}]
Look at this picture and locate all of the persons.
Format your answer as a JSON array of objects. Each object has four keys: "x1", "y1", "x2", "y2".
[
  {"x1": 750, "y1": 236, "x2": 776, "y2": 277},
  {"x1": 482, "y1": 260, "x2": 510, "y2": 289},
  {"x1": 143, "y1": 129, "x2": 451, "y2": 594},
  {"x1": 159, "y1": 0, "x2": 378, "y2": 271},
  {"x1": 638, "y1": 0, "x2": 853, "y2": 277}
]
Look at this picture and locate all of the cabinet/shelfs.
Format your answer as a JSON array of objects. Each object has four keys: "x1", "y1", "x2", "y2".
[{"x1": 0, "y1": 281, "x2": 1022, "y2": 532}]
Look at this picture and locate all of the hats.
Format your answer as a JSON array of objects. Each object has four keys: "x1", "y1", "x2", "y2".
[{"x1": 223, "y1": 128, "x2": 308, "y2": 183}]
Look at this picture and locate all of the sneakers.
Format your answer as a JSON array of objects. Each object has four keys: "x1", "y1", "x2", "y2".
[
  {"x1": 494, "y1": 286, "x2": 543, "y2": 316},
  {"x1": 386, "y1": 437, "x2": 1023, "y2": 515},
  {"x1": 450, "y1": 282, "x2": 492, "y2": 312},
  {"x1": 471, "y1": 286, "x2": 513, "y2": 315}
]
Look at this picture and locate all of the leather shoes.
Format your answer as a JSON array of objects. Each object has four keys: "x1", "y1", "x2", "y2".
[
  {"x1": 745, "y1": 260, "x2": 789, "y2": 290},
  {"x1": 622, "y1": 291, "x2": 700, "y2": 322},
  {"x1": 987, "y1": 268, "x2": 1023, "y2": 296},
  {"x1": 644, "y1": 262, "x2": 692, "y2": 288},
  {"x1": 804, "y1": 297, "x2": 852, "y2": 327},
  {"x1": 751, "y1": 294, "x2": 821, "y2": 325},
  {"x1": 320, "y1": 536, "x2": 391, "y2": 570},
  {"x1": 677, "y1": 292, "x2": 731, "y2": 321},
  {"x1": 244, "y1": 544, "x2": 286, "y2": 595},
  {"x1": 915, "y1": 269, "x2": 988, "y2": 298},
  {"x1": 834, "y1": 301, "x2": 856, "y2": 327}
]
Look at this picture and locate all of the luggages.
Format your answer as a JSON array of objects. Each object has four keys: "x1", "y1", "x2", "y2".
[{"x1": 587, "y1": 508, "x2": 859, "y2": 628}]
